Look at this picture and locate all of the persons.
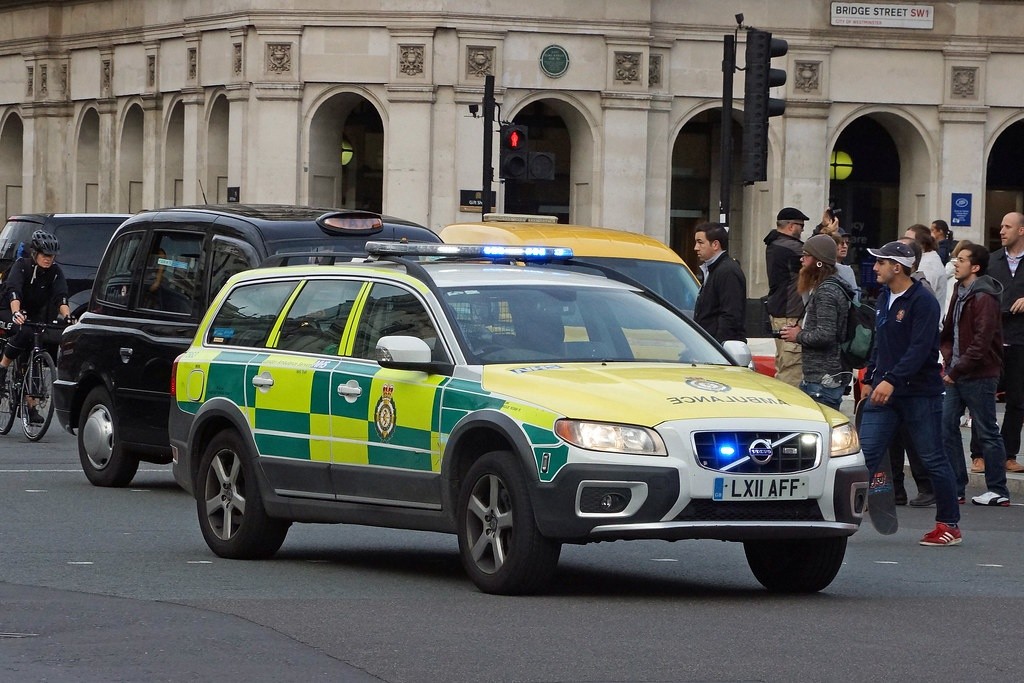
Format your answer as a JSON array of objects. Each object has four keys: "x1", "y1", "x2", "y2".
[
  {"x1": 780, "y1": 234, "x2": 852, "y2": 411},
  {"x1": 763, "y1": 208, "x2": 974, "y2": 507},
  {"x1": 970, "y1": 212, "x2": 1024, "y2": 473},
  {"x1": 942, "y1": 244, "x2": 1010, "y2": 506},
  {"x1": 140, "y1": 247, "x2": 173, "y2": 312},
  {"x1": 858, "y1": 241, "x2": 965, "y2": 546},
  {"x1": 1, "y1": 229, "x2": 78, "y2": 427},
  {"x1": 693, "y1": 222, "x2": 746, "y2": 348}
]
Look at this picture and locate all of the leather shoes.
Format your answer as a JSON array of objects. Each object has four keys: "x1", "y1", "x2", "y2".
[
  {"x1": 894, "y1": 491, "x2": 907, "y2": 505},
  {"x1": 909, "y1": 491, "x2": 936, "y2": 507}
]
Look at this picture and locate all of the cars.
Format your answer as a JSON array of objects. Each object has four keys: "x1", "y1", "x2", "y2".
[
  {"x1": 168, "y1": 237, "x2": 873, "y2": 598},
  {"x1": 0, "y1": 211, "x2": 136, "y2": 367},
  {"x1": 52, "y1": 201, "x2": 444, "y2": 487}
]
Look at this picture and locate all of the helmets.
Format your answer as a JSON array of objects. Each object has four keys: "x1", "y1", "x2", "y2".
[{"x1": 31, "y1": 230, "x2": 61, "y2": 255}]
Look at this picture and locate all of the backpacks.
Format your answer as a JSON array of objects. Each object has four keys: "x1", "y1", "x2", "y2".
[{"x1": 816, "y1": 282, "x2": 877, "y2": 369}]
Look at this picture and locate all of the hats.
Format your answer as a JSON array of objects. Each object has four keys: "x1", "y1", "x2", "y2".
[
  {"x1": 838, "y1": 228, "x2": 852, "y2": 238},
  {"x1": 867, "y1": 242, "x2": 915, "y2": 268},
  {"x1": 777, "y1": 208, "x2": 810, "y2": 220},
  {"x1": 802, "y1": 234, "x2": 838, "y2": 265}
]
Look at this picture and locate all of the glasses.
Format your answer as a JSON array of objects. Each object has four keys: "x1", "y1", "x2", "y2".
[
  {"x1": 40, "y1": 251, "x2": 52, "y2": 257},
  {"x1": 841, "y1": 242, "x2": 850, "y2": 246},
  {"x1": 788, "y1": 222, "x2": 805, "y2": 229}
]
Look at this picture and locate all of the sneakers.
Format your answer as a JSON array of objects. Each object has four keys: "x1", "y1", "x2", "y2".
[
  {"x1": 1007, "y1": 460, "x2": 1024, "y2": 471},
  {"x1": 17, "y1": 405, "x2": 44, "y2": 423},
  {"x1": 918, "y1": 522, "x2": 962, "y2": 546},
  {"x1": 972, "y1": 458, "x2": 985, "y2": 472},
  {"x1": 957, "y1": 497, "x2": 966, "y2": 503},
  {"x1": 972, "y1": 491, "x2": 1010, "y2": 507},
  {"x1": 0, "y1": 366, "x2": 8, "y2": 391}
]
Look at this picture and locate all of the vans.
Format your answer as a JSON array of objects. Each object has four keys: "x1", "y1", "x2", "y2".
[{"x1": 438, "y1": 212, "x2": 705, "y2": 323}]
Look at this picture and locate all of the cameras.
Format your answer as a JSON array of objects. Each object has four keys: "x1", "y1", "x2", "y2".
[{"x1": 772, "y1": 325, "x2": 796, "y2": 339}]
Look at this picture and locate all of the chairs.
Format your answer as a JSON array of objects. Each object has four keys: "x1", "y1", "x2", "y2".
[
  {"x1": 510, "y1": 298, "x2": 569, "y2": 359},
  {"x1": 471, "y1": 299, "x2": 499, "y2": 350}
]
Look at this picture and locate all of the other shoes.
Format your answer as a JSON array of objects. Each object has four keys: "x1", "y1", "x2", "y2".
[{"x1": 960, "y1": 415, "x2": 972, "y2": 427}]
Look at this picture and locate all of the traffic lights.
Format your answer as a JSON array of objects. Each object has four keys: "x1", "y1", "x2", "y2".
[
  {"x1": 766, "y1": 36, "x2": 788, "y2": 119},
  {"x1": 499, "y1": 120, "x2": 530, "y2": 180}
]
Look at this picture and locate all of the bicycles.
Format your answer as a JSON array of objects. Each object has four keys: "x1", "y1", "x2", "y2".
[{"x1": 0, "y1": 313, "x2": 77, "y2": 442}]
